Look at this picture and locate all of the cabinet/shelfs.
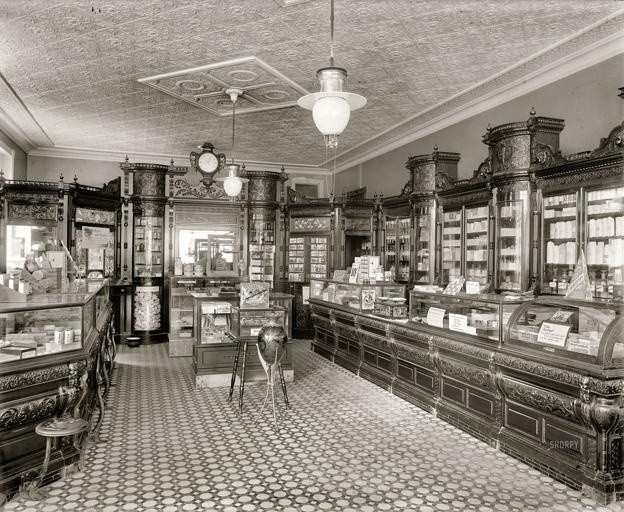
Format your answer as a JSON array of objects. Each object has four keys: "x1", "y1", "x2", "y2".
[
  {"x1": 119, "y1": 153, "x2": 333, "y2": 388},
  {"x1": 305, "y1": 277, "x2": 623, "y2": 503},
  {"x1": 374, "y1": 84, "x2": 624, "y2": 304},
  {"x1": 0, "y1": 169, "x2": 120, "y2": 498}
]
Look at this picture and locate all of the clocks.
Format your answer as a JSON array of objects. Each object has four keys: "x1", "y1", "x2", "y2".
[{"x1": 190, "y1": 141, "x2": 226, "y2": 187}]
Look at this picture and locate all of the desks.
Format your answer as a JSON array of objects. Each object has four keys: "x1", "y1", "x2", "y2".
[{"x1": 223, "y1": 332, "x2": 293, "y2": 414}]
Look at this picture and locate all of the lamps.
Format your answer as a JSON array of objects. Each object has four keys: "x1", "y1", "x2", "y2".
[
  {"x1": 216, "y1": 88, "x2": 250, "y2": 207},
  {"x1": 296, "y1": 1, "x2": 368, "y2": 209}
]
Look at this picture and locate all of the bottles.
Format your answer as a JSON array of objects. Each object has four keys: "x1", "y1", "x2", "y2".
[
  {"x1": 54, "y1": 327, "x2": 81, "y2": 345},
  {"x1": 9, "y1": 273, "x2": 33, "y2": 296},
  {"x1": 173, "y1": 257, "x2": 182, "y2": 276}
]
